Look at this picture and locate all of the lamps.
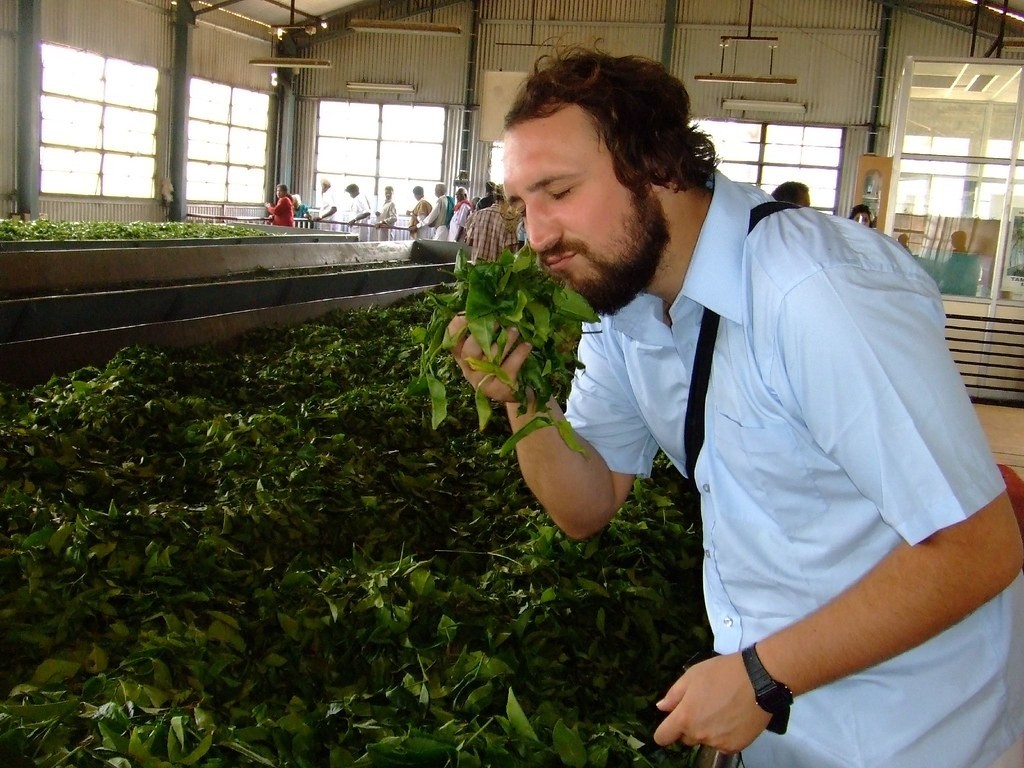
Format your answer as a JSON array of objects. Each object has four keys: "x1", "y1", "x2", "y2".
[
  {"x1": 347, "y1": 82, "x2": 416, "y2": 95},
  {"x1": 693, "y1": 44, "x2": 798, "y2": 85},
  {"x1": 350, "y1": 19, "x2": 463, "y2": 37},
  {"x1": 249, "y1": 59, "x2": 331, "y2": 68},
  {"x1": 721, "y1": 99, "x2": 807, "y2": 114}
]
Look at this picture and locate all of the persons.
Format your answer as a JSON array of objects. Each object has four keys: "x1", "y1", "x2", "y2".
[
  {"x1": 406, "y1": 181, "x2": 527, "y2": 264},
  {"x1": 372, "y1": 186, "x2": 398, "y2": 241},
  {"x1": 848, "y1": 205, "x2": 871, "y2": 228},
  {"x1": 771, "y1": 182, "x2": 810, "y2": 207},
  {"x1": 264, "y1": 185, "x2": 294, "y2": 228},
  {"x1": 445, "y1": 53, "x2": 1024, "y2": 768},
  {"x1": 289, "y1": 194, "x2": 311, "y2": 220},
  {"x1": 344, "y1": 184, "x2": 371, "y2": 242},
  {"x1": 939, "y1": 231, "x2": 968, "y2": 295},
  {"x1": 311, "y1": 180, "x2": 338, "y2": 231}
]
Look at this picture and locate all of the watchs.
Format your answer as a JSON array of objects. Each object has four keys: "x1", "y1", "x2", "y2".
[{"x1": 742, "y1": 643, "x2": 794, "y2": 714}]
